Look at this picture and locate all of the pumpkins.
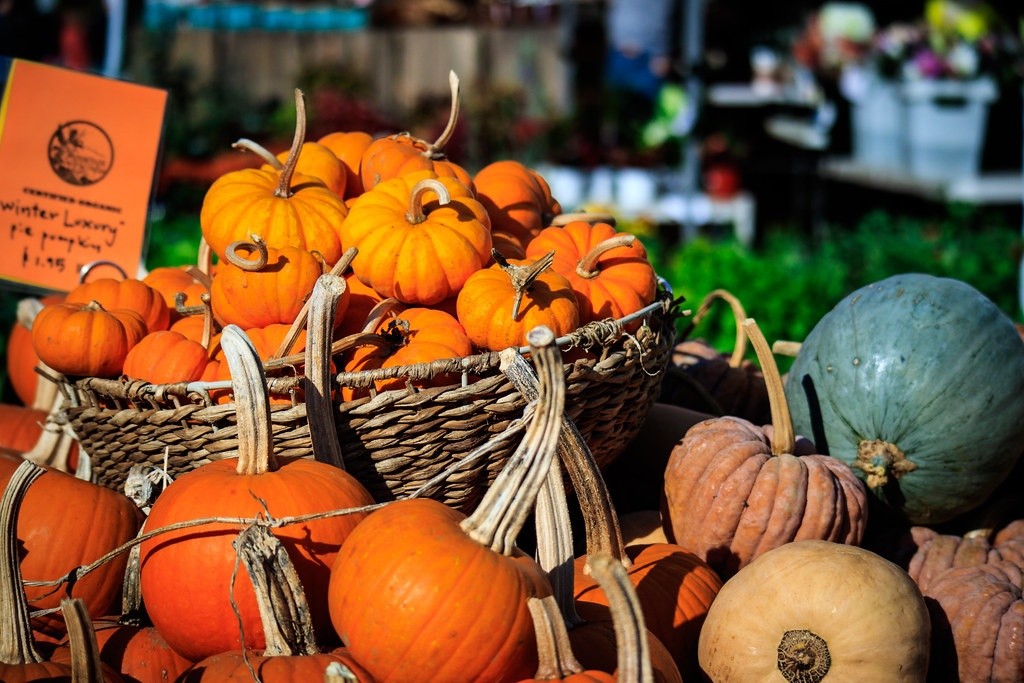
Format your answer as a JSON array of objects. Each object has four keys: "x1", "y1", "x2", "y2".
[{"x1": 0, "y1": 69, "x2": 1024, "y2": 683}]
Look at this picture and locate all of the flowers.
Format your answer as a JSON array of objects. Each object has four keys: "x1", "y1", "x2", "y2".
[{"x1": 789, "y1": 0, "x2": 991, "y2": 79}]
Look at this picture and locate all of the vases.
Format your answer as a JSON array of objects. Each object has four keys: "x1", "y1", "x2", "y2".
[{"x1": 839, "y1": 71, "x2": 1002, "y2": 190}]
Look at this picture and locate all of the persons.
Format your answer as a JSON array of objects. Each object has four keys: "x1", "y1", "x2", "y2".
[
  {"x1": 658, "y1": 1, "x2": 1024, "y2": 246},
  {"x1": 562, "y1": 0, "x2": 673, "y2": 165}
]
